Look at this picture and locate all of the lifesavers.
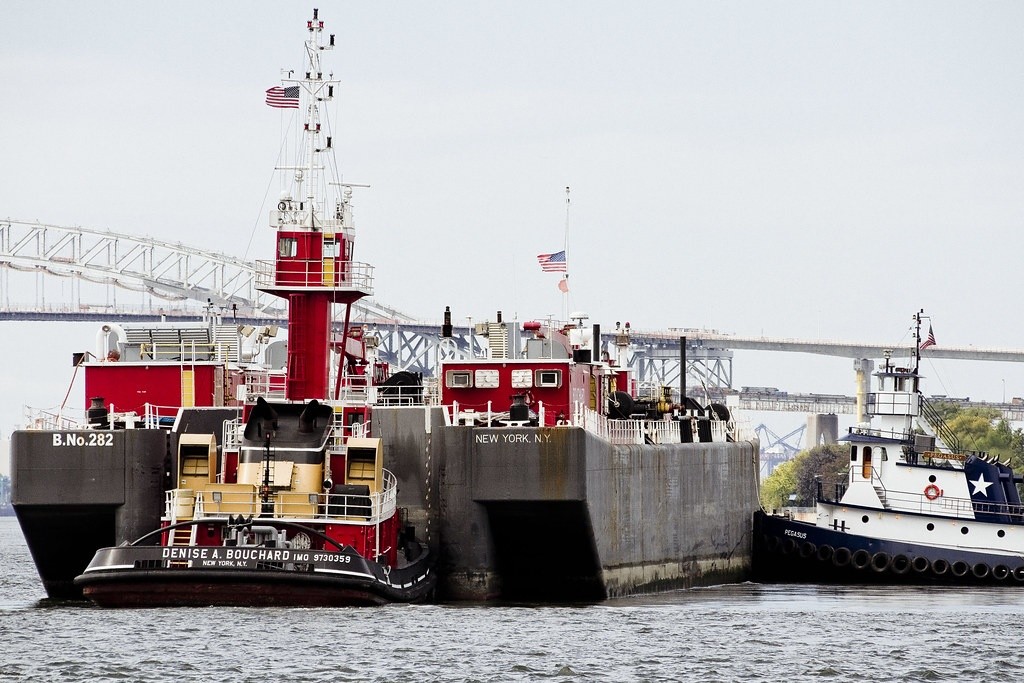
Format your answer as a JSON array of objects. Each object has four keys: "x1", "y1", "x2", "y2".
[
  {"x1": 518, "y1": 390, "x2": 536, "y2": 407},
  {"x1": 767, "y1": 535, "x2": 1024, "y2": 583},
  {"x1": 924, "y1": 484, "x2": 940, "y2": 500},
  {"x1": 277, "y1": 201, "x2": 286, "y2": 210}
]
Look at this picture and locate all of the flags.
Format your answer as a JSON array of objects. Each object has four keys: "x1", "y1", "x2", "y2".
[
  {"x1": 536, "y1": 250, "x2": 566, "y2": 273},
  {"x1": 265, "y1": 85, "x2": 300, "y2": 109},
  {"x1": 920, "y1": 322, "x2": 936, "y2": 350}
]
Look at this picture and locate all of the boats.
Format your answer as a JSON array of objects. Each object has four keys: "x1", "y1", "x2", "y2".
[
  {"x1": 71, "y1": 5, "x2": 435, "y2": 609},
  {"x1": 2, "y1": 283, "x2": 765, "y2": 607},
  {"x1": 758, "y1": 306, "x2": 1024, "y2": 583}
]
